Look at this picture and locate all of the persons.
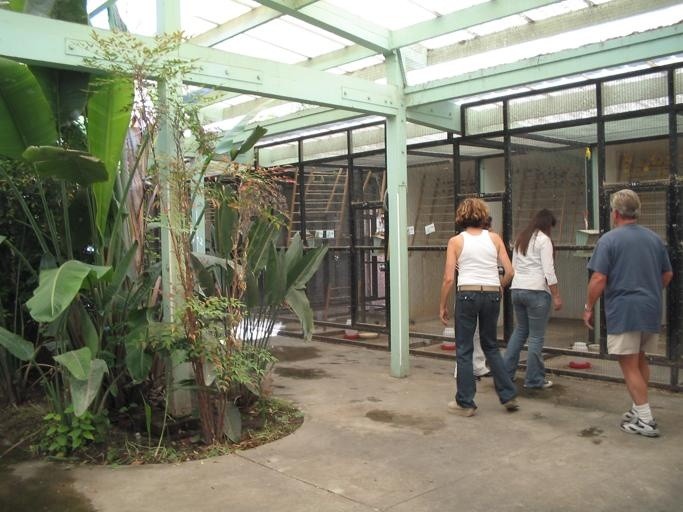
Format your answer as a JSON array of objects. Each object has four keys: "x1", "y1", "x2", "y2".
[
  {"x1": 453, "y1": 216, "x2": 493, "y2": 380},
  {"x1": 580, "y1": 188, "x2": 674, "y2": 438},
  {"x1": 502, "y1": 207, "x2": 563, "y2": 390},
  {"x1": 437, "y1": 197, "x2": 520, "y2": 417}
]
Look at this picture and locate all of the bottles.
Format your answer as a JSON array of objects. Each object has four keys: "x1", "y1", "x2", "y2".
[
  {"x1": 344, "y1": 319, "x2": 358, "y2": 337},
  {"x1": 443, "y1": 327, "x2": 456, "y2": 348},
  {"x1": 572, "y1": 341, "x2": 588, "y2": 365}
]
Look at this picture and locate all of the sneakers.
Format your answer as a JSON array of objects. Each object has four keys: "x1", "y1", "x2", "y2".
[
  {"x1": 622, "y1": 409, "x2": 638, "y2": 422},
  {"x1": 620, "y1": 418, "x2": 660, "y2": 439},
  {"x1": 448, "y1": 401, "x2": 475, "y2": 417},
  {"x1": 505, "y1": 399, "x2": 519, "y2": 411},
  {"x1": 524, "y1": 380, "x2": 552, "y2": 388}
]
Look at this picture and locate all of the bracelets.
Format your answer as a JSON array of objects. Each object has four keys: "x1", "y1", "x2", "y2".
[{"x1": 583, "y1": 302, "x2": 594, "y2": 313}]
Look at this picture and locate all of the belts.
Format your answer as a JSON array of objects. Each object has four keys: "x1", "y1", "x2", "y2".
[{"x1": 458, "y1": 285, "x2": 500, "y2": 292}]
